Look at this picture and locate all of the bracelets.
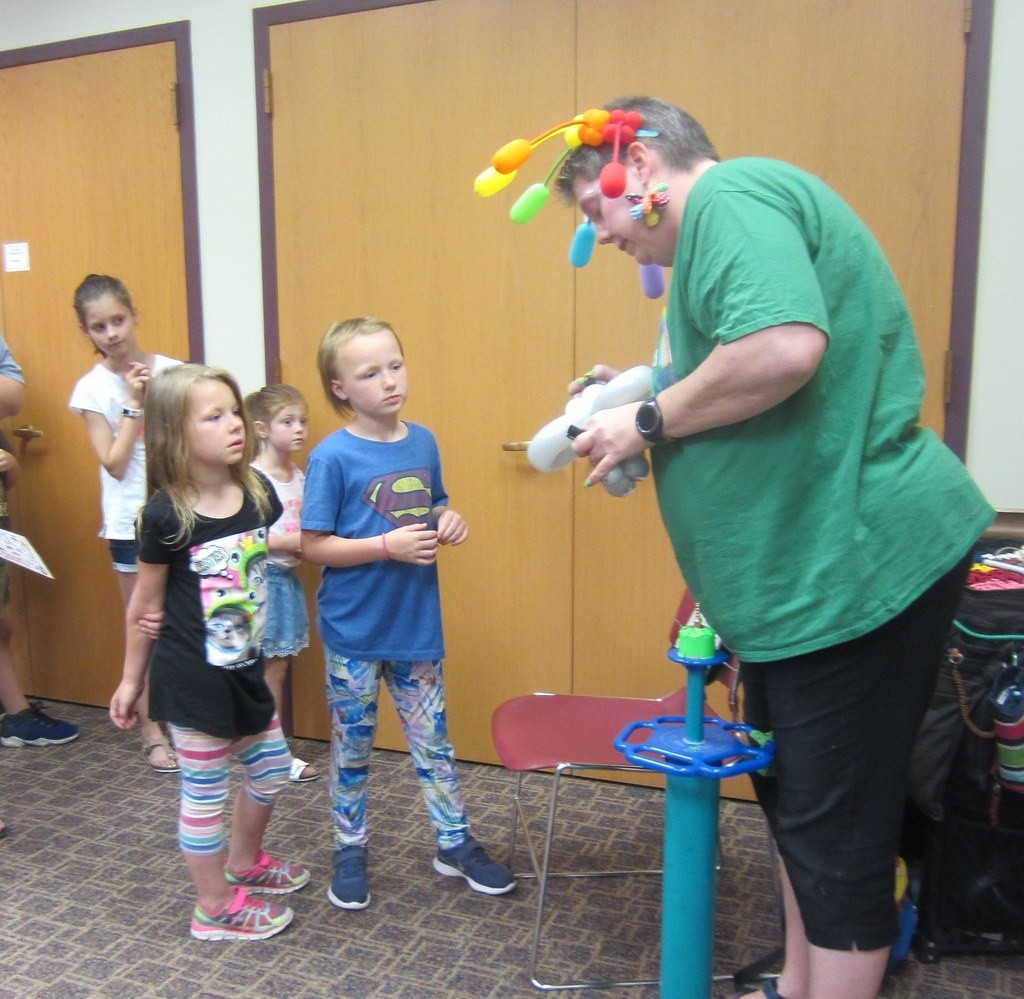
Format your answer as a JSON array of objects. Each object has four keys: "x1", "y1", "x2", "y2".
[{"x1": 382, "y1": 532, "x2": 391, "y2": 562}]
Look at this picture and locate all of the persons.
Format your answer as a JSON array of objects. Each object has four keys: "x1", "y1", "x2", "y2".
[
  {"x1": 108, "y1": 363, "x2": 310, "y2": 942},
  {"x1": 552, "y1": 91, "x2": 997, "y2": 999},
  {"x1": 0, "y1": 332, "x2": 81, "y2": 749},
  {"x1": 243, "y1": 383, "x2": 321, "y2": 782},
  {"x1": 299, "y1": 314, "x2": 516, "y2": 909},
  {"x1": 69, "y1": 273, "x2": 186, "y2": 772}
]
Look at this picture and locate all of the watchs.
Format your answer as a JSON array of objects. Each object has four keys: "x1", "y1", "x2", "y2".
[
  {"x1": 635, "y1": 397, "x2": 675, "y2": 445},
  {"x1": 122, "y1": 406, "x2": 141, "y2": 417}
]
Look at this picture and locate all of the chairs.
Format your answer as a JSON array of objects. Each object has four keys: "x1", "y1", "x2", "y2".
[{"x1": 492, "y1": 582, "x2": 787, "y2": 989}]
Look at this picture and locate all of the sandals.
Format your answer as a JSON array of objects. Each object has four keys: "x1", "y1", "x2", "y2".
[
  {"x1": 730, "y1": 977, "x2": 786, "y2": 999},
  {"x1": 142, "y1": 739, "x2": 181, "y2": 773},
  {"x1": 289, "y1": 758, "x2": 321, "y2": 782}
]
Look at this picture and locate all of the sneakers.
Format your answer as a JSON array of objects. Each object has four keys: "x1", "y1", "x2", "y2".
[
  {"x1": 0, "y1": 701, "x2": 81, "y2": 748},
  {"x1": 223, "y1": 849, "x2": 311, "y2": 895},
  {"x1": 327, "y1": 846, "x2": 372, "y2": 909},
  {"x1": 432, "y1": 835, "x2": 517, "y2": 896},
  {"x1": 191, "y1": 885, "x2": 294, "y2": 941}
]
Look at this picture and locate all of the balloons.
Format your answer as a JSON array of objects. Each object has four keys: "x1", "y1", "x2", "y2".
[
  {"x1": 526, "y1": 363, "x2": 653, "y2": 498},
  {"x1": 474, "y1": 107, "x2": 664, "y2": 301}
]
